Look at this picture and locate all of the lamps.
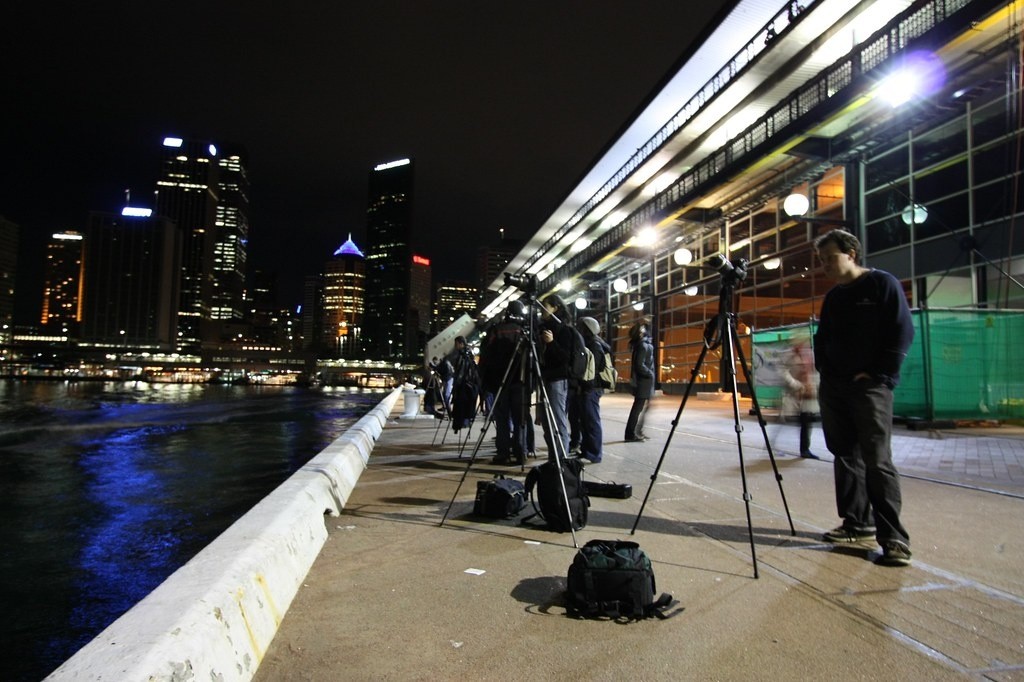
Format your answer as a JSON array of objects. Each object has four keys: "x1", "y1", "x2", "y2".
[
  {"x1": 783, "y1": 193, "x2": 854, "y2": 229},
  {"x1": 683, "y1": 286, "x2": 699, "y2": 297},
  {"x1": 901, "y1": 203, "x2": 928, "y2": 226},
  {"x1": 763, "y1": 256, "x2": 781, "y2": 270},
  {"x1": 574, "y1": 297, "x2": 609, "y2": 313},
  {"x1": 632, "y1": 300, "x2": 644, "y2": 311},
  {"x1": 614, "y1": 278, "x2": 656, "y2": 298},
  {"x1": 674, "y1": 248, "x2": 714, "y2": 269}
]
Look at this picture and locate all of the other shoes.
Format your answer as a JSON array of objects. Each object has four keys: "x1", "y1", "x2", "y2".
[
  {"x1": 636, "y1": 433, "x2": 650, "y2": 440},
  {"x1": 568, "y1": 446, "x2": 581, "y2": 456},
  {"x1": 574, "y1": 456, "x2": 594, "y2": 464},
  {"x1": 800, "y1": 451, "x2": 818, "y2": 459},
  {"x1": 493, "y1": 455, "x2": 509, "y2": 465},
  {"x1": 625, "y1": 435, "x2": 642, "y2": 442},
  {"x1": 516, "y1": 454, "x2": 528, "y2": 464}
]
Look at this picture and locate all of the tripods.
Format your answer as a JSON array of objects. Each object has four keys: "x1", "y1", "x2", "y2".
[
  {"x1": 411, "y1": 329, "x2": 516, "y2": 466},
  {"x1": 632, "y1": 277, "x2": 796, "y2": 579},
  {"x1": 438, "y1": 295, "x2": 579, "y2": 548}
]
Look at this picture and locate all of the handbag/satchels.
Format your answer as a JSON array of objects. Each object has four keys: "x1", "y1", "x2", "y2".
[
  {"x1": 524, "y1": 459, "x2": 590, "y2": 531},
  {"x1": 473, "y1": 474, "x2": 526, "y2": 522}
]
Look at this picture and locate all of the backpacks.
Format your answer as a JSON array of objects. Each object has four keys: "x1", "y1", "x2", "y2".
[{"x1": 567, "y1": 539, "x2": 673, "y2": 620}]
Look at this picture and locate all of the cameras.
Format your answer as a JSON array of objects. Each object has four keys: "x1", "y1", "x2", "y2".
[
  {"x1": 708, "y1": 253, "x2": 749, "y2": 282},
  {"x1": 502, "y1": 271, "x2": 540, "y2": 295}
]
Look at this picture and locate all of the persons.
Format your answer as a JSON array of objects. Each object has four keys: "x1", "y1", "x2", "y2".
[
  {"x1": 428, "y1": 293, "x2": 611, "y2": 466},
  {"x1": 624, "y1": 322, "x2": 655, "y2": 442},
  {"x1": 813, "y1": 229, "x2": 916, "y2": 565},
  {"x1": 782, "y1": 336, "x2": 820, "y2": 460}
]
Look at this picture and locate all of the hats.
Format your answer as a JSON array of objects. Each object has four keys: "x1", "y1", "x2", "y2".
[{"x1": 581, "y1": 317, "x2": 601, "y2": 336}]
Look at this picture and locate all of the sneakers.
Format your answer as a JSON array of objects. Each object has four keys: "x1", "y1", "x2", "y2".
[
  {"x1": 883, "y1": 543, "x2": 911, "y2": 566},
  {"x1": 824, "y1": 527, "x2": 877, "y2": 543}
]
